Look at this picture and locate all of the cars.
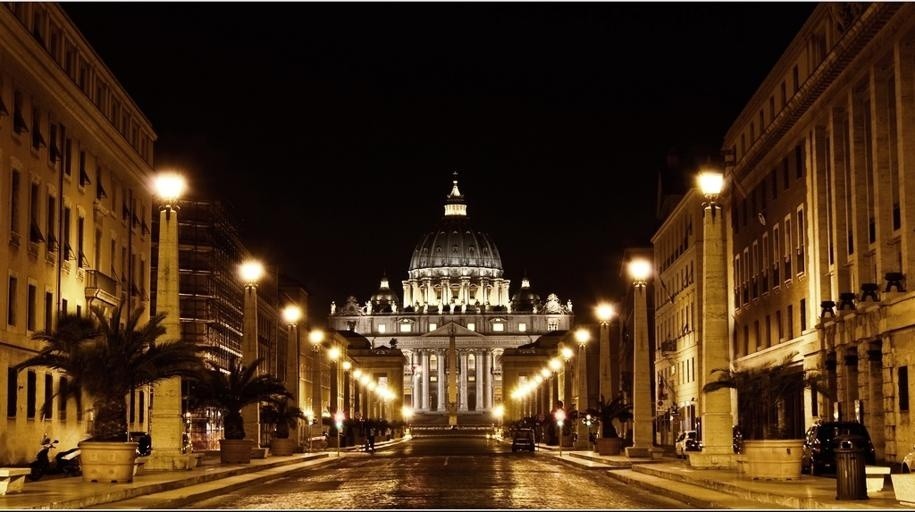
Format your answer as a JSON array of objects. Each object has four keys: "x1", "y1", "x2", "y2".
[
  {"x1": 675, "y1": 431, "x2": 697, "y2": 459},
  {"x1": 804, "y1": 420, "x2": 876, "y2": 474},
  {"x1": 901, "y1": 445, "x2": 915, "y2": 473},
  {"x1": 129, "y1": 431, "x2": 150, "y2": 453},
  {"x1": 512, "y1": 428, "x2": 535, "y2": 453},
  {"x1": 183, "y1": 432, "x2": 193, "y2": 453}
]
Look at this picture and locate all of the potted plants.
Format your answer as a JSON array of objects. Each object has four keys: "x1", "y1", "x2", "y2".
[
  {"x1": 260, "y1": 391, "x2": 308, "y2": 455},
  {"x1": 188, "y1": 354, "x2": 288, "y2": 467},
  {"x1": 560, "y1": 391, "x2": 633, "y2": 456},
  {"x1": 701, "y1": 349, "x2": 838, "y2": 482},
  {"x1": 12, "y1": 293, "x2": 203, "y2": 485}
]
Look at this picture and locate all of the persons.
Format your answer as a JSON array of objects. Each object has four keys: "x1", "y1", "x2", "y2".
[{"x1": 368, "y1": 431, "x2": 376, "y2": 454}]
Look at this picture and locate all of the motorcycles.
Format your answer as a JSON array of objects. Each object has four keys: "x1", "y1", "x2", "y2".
[{"x1": 27, "y1": 432, "x2": 82, "y2": 481}]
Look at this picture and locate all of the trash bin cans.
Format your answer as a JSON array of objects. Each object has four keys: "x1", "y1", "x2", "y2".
[
  {"x1": 834, "y1": 435, "x2": 872, "y2": 500},
  {"x1": 562, "y1": 427, "x2": 573, "y2": 446}
]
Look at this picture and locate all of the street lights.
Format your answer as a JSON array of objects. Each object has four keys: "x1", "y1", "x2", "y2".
[
  {"x1": 308, "y1": 411, "x2": 342, "y2": 456},
  {"x1": 555, "y1": 408, "x2": 565, "y2": 456}
]
